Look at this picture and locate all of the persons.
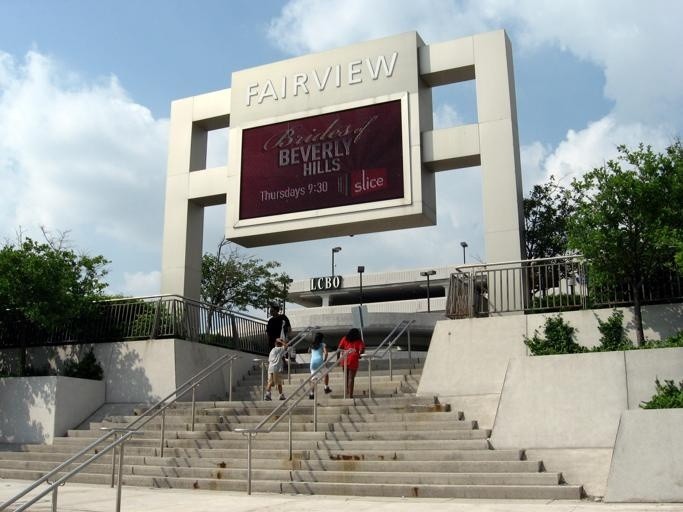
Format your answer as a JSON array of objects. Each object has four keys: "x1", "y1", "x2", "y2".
[
  {"x1": 336, "y1": 328, "x2": 365, "y2": 399},
  {"x1": 264, "y1": 338, "x2": 288, "y2": 400},
  {"x1": 308, "y1": 332, "x2": 332, "y2": 400},
  {"x1": 265, "y1": 305, "x2": 291, "y2": 372}
]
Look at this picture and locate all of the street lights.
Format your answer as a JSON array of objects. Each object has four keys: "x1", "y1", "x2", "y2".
[
  {"x1": 358, "y1": 265, "x2": 364, "y2": 306},
  {"x1": 331, "y1": 246, "x2": 341, "y2": 277},
  {"x1": 420, "y1": 270, "x2": 438, "y2": 312},
  {"x1": 460, "y1": 241, "x2": 469, "y2": 263}
]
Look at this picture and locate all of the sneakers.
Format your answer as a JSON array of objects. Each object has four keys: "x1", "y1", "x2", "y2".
[
  {"x1": 279, "y1": 393, "x2": 286, "y2": 399},
  {"x1": 310, "y1": 395, "x2": 314, "y2": 399},
  {"x1": 265, "y1": 394, "x2": 272, "y2": 400},
  {"x1": 324, "y1": 387, "x2": 331, "y2": 393}
]
